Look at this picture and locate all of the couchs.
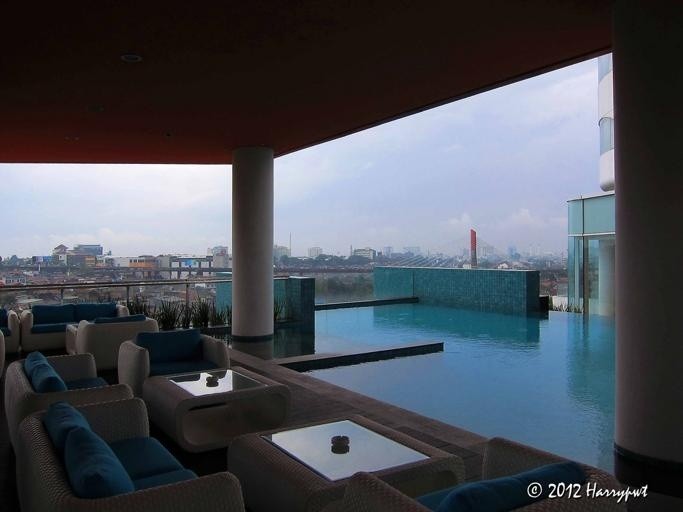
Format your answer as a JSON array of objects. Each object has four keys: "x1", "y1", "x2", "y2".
[{"x1": 0, "y1": 301, "x2": 629, "y2": 512}]
